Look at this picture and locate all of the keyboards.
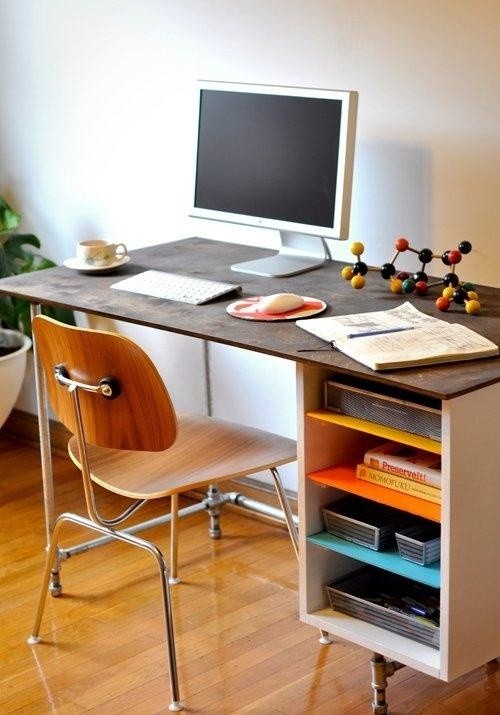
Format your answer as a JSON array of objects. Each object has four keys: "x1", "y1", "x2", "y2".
[{"x1": 110, "y1": 269, "x2": 242, "y2": 305}]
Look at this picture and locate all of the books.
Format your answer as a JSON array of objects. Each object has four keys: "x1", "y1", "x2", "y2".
[
  {"x1": 362, "y1": 441, "x2": 442, "y2": 491},
  {"x1": 355, "y1": 463, "x2": 442, "y2": 506},
  {"x1": 296, "y1": 300, "x2": 500, "y2": 372}
]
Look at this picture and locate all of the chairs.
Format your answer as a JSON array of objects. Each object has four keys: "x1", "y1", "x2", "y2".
[{"x1": 27, "y1": 313, "x2": 334, "y2": 712}]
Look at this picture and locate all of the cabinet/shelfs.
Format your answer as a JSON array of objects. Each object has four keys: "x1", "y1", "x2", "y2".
[{"x1": 294, "y1": 361, "x2": 500, "y2": 685}]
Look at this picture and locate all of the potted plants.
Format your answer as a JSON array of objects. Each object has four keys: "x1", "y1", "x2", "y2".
[{"x1": 0, "y1": 195, "x2": 76, "y2": 434}]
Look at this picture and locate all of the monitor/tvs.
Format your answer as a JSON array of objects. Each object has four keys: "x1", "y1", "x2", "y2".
[{"x1": 187, "y1": 79, "x2": 359, "y2": 279}]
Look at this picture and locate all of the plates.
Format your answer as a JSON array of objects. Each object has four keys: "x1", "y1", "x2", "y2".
[{"x1": 63, "y1": 255, "x2": 131, "y2": 275}]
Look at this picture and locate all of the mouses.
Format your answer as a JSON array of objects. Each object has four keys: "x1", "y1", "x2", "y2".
[{"x1": 258, "y1": 293, "x2": 304, "y2": 315}]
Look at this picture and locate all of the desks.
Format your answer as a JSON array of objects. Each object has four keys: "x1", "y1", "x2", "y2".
[{"x1": 0, "y1": 236, "x2": 500, "y2": 715}]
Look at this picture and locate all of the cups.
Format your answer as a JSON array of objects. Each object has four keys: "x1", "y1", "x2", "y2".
[{"x1": 76, "y1": 240, "x2": 128, "y2": 266}]
